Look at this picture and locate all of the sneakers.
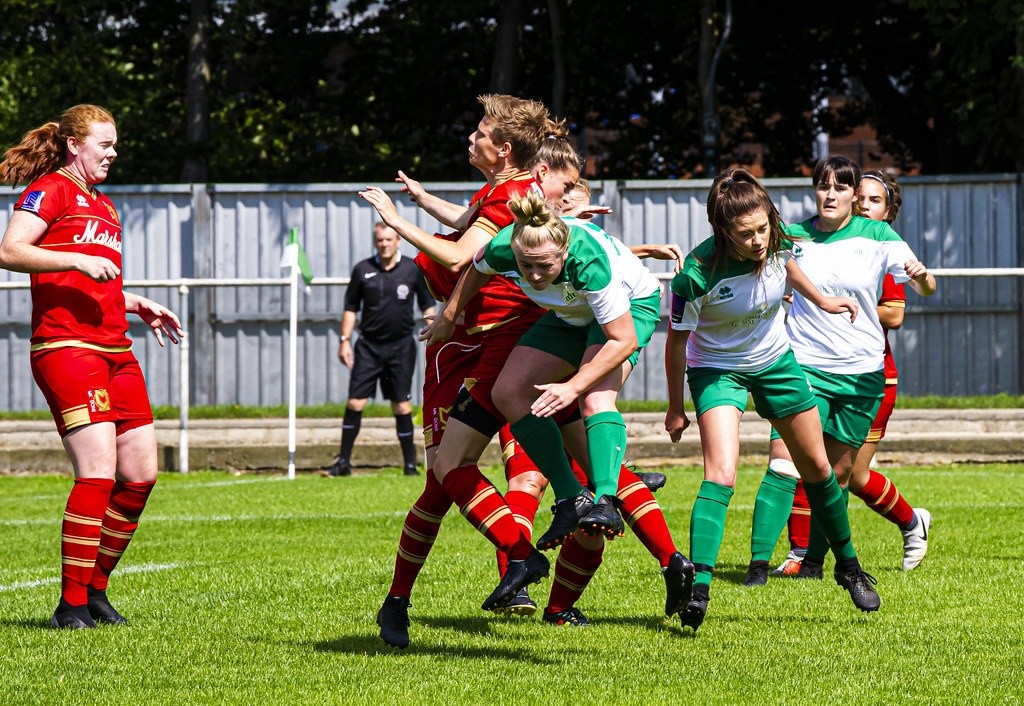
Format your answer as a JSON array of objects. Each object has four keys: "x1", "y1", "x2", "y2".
[
  {"x1": 319, "y1": 459, "x2": 351, "y2": 478},
  {"x1": 624, "y1": 460, "x2": 667, "y2": 493},
  {"x1": 542, "y1": 607, "x2": 589, "y2": 627},
  {"x1": 481, "y1": 552, "x2": 550, "y2": 611},
  {"x1": 798, "y1": 559, "x2": 824, "y2": 580},
  {"x1": 405, "y1": 464, "x2": 419, "y2": 476},
  {"x1": 680, "y1": 587, "x2": 710, "y2": 632},
  {"x1": 770, "y1": 550, "x2": 806, "y2": 578},
  {"x1": 902, "y1": 508, "x2": 932, "y2": 572},
  {"x1": 536, "y1": 488, "x2": 595, "y2": 551},
  {"x1": 87, "y1": 592, "x2": 132, "y2": 627},
  {"x1": 377, "y1": 593, "x2": 413, "y2": 650},
  {"x1": 663, "y1": 552, "x2": 697, "y2": 617},
  {"x1": 744, "y1": 560, "x2": 768, "y2": 586},
  {"x1": 578, "y1": 495, "x2": 625, "y2": 541},
  {"x1": 833, "y1": 558, "x2": 880, "y2": 613},
  {"x1": 50, "y1": 598, "x2": 100, "y2": 631},
  {"x1": 504, "y1": 587, "x2": 537, "y2": 616}
]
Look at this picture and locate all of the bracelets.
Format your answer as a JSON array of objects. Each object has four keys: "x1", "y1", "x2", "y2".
[{"x1": 340, "y1": 336, "x2": 350, "y2": 342}]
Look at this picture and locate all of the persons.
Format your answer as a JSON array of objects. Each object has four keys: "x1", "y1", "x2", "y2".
[
  {"x1": 0, "y1": 104, "x2": 184, "y2": 629},
  {"x1": 319, "y1": 221, "x2": 437, "y2": 478},
  {"x1": 665, "y1": 168, "x2": 881, "y2": 629},
  {"x1": 357, "y1": 93, "x2": 696, "y2": 649},
  {"x1": 774, "y1": 170, "x2": 933, "y2": 576},
  {"x1": 745, "y1": 156, "x2": 937, "y2": 588},
  {"x1": 418, "y1": 192, "x2": 660, "y2": 551}
]
